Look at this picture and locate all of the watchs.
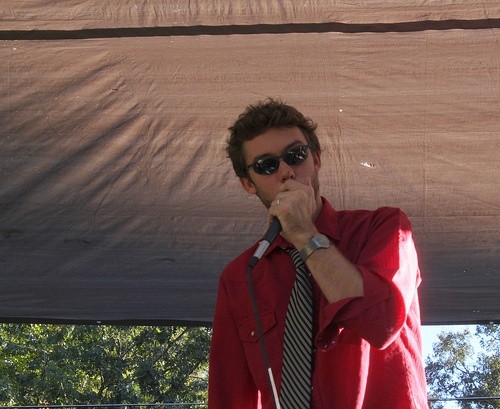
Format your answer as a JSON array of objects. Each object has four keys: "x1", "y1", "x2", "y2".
[{"x1": 298, "y1": 231, "x2": 331, "y2": 262}]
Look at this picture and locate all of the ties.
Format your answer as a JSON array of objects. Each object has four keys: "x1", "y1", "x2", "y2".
[{"x1": 278, "y1": 247, "x2": 314, "y2": 409}]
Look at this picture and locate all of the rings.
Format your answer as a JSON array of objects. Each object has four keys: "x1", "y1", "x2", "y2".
[{"x1": 276, "y1": 198, "x2": 280, "y2": 205}]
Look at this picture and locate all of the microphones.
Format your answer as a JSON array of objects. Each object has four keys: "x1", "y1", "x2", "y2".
[{"x1": 246, "y1": 217, "x2": 282, "y2": 268}]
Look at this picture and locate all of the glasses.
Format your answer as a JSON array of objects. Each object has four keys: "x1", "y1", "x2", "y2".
[{"x1": 244, "y1": 144, "x2": 311, "y2": 176}]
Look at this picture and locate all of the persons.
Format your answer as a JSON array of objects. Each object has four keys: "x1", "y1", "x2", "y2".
[{"x1": 208, "y1": 97, "x2": 431, "y2": 409}]
